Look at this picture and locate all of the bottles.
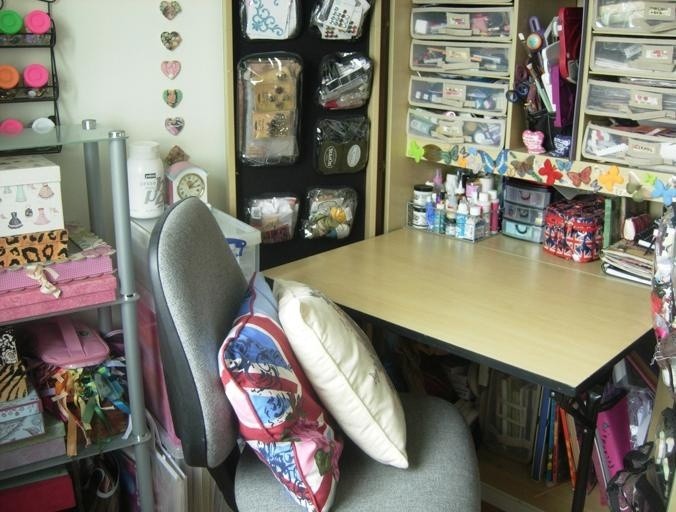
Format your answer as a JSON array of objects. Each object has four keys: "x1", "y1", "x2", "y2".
[
  {"x1": 127, "y1": 141, "x2": 165, "y2": 219},
  {"x1": 412, "y1": 171, "x2": 499, "y2": 240}
]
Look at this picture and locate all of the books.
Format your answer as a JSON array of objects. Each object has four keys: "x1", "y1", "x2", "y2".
[
  {"x1": 493, "y1": 376, "x2": 598, "y2": 494},
  {"x1": 599, "y1": 225, "x2": 661, "y2": 286}
]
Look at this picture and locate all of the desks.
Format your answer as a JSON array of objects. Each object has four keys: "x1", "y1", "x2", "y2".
[{"x1": 262, "y1": 223, "x2": 666, "y2": 511}]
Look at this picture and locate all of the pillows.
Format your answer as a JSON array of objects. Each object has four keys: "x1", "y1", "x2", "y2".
[
  {"x1": 216, "y1": 268, "x2": 350, "y2": 512},
  {"x1": 266, "y1": 276, "x2": 413, "y2": 472}
]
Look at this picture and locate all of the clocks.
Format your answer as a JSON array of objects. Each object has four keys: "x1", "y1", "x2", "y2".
[{"x1": 166, "y1": 161, "x2": 209, "y2": 205}]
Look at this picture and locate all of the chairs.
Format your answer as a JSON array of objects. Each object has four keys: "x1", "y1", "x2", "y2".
[{"x1": 148, "y1": 196, "x2": 492, "y2": 511}]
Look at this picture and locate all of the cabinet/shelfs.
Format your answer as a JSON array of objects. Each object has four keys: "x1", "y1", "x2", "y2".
[
  {"x1": 385, "y1": 2, "x2": 676, "y2": 258},
  {"x1": 0, "y1": 120, "x2": 159, "y2": 512}
]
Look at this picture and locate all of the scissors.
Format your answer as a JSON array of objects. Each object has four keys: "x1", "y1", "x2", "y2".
[{"x1": 505, "y1": 63, "x2": 529, "y2": 104}]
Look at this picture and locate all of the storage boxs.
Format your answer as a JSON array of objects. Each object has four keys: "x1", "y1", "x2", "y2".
[
  {"x1": 0, "y1": 225, "x2": 70, "y2": 273},
  {"x1": 129, "y1": 211, "x2": 260, "y2": 309},
  {"x1": 30, "y1": 323, "x2": 108, "y2": 365},
  {"x1": 576, "y1": 1, "x2": 674, "y2": 173},
  {"x1": 504, "y1": 187, "x2": 603, "y2": 258},
  {"x1": 409, "y1": 7, "x2": 514, "y2": 151},
  {"x1": 0, "y1": 148, "x2": 69, "y2": 231},
  {"x1": 0, "y1": 328, "x2": 81, "y2": 511}
]
